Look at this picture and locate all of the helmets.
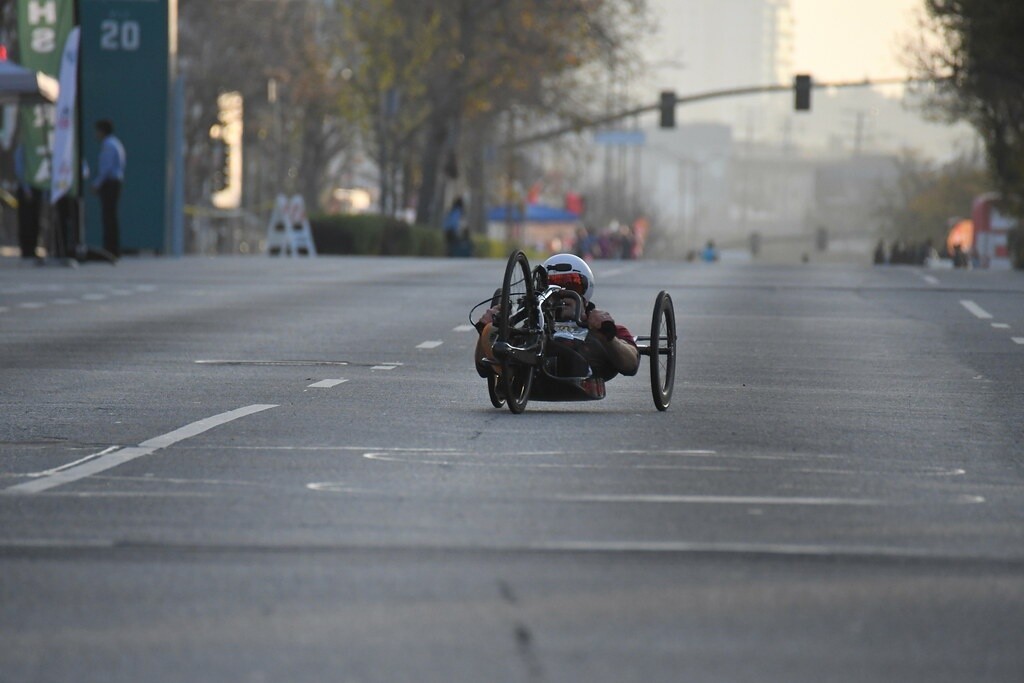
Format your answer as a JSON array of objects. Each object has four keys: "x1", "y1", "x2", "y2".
[{"x1": 541, "y1": 254, "x2": 595, "y2": 306}]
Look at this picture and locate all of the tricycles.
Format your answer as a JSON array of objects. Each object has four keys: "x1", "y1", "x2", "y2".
[{"x1": 468, "y1": 248, "x2": 678, "y2": 415}]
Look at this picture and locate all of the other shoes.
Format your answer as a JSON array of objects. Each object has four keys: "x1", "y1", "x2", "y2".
[{"x1": 482, "y1": 322, "x2": 505, "y2": 380}]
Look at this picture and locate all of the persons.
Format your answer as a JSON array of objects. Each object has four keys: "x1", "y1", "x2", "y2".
[
  {"x1": 15, "y1": 142, "x2": 44, "y2": 258},
  {"x1": 873, "y1": 231, "x2": 1024, "y2": 273},
  {"x1": 440, "y1": 194, "x2": 477, "y2": 259},
  {"x1": 570, "y1": 225, "x2": 643, "y2": 263},
  {"x1": 701, "y1": 238, "x2": 718, "y2": 265},
  {"x1": 479, "y1": 253, "x2": 642, "y2": 382},
  {"x1": 91, "y1": 120, "x2": 126, "y2": 258}
]
